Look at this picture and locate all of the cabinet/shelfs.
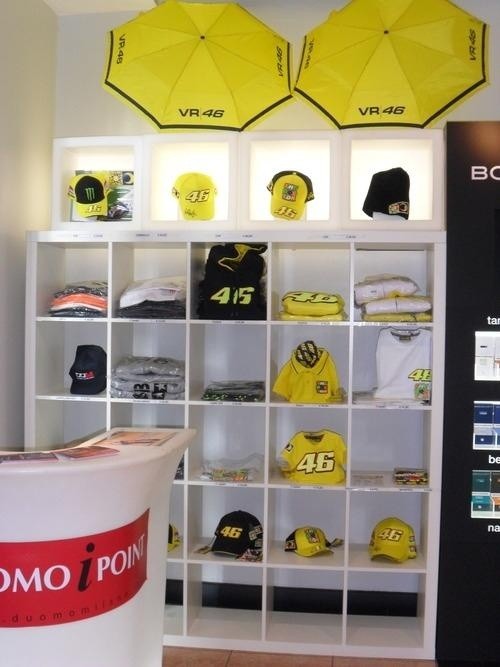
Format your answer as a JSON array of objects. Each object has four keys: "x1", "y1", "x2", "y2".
[
  {"x1": 51, "y1": 129, "x2": 447, "y2": 230},
  {"x1": 23, "y1": 230, "x2": 447, "y2": 659}
]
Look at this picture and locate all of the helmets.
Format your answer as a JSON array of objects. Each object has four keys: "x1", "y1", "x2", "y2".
[{"x1": 367, "y1": 516, "x2": 417, "y2": 563}]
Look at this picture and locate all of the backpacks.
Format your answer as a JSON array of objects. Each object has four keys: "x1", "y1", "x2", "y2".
[
  {"x1": 68, "y1": 345, "x2": 108, "y2": 395},
  {"x1": 196, "y1": 242, "x2": 266, "y2": 320}
]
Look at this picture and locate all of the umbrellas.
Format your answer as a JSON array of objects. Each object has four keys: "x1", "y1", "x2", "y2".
[
  {"x1": 291, "y1": 0, "x2": 491, "y2": 129},
  {"x1": 102, "y1": 1, "x2": 297, "y2": 132}
]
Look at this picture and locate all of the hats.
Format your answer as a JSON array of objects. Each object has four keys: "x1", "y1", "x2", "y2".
[
  {"x1": 172, "y1": 171, "x2": 217, "y2": 221},
  {"x1": 266, "y1": 170, "x2": 315, "y2": 219},
  {"x1": 211, "y1": 510, "x2": 263, "y2": 557},
  {"x1": 285, "y1": 526, "x2": 334, "y2": 556},
  {"x1": 65, "y1": 172, "x2": 112, "y2": 218}
]
